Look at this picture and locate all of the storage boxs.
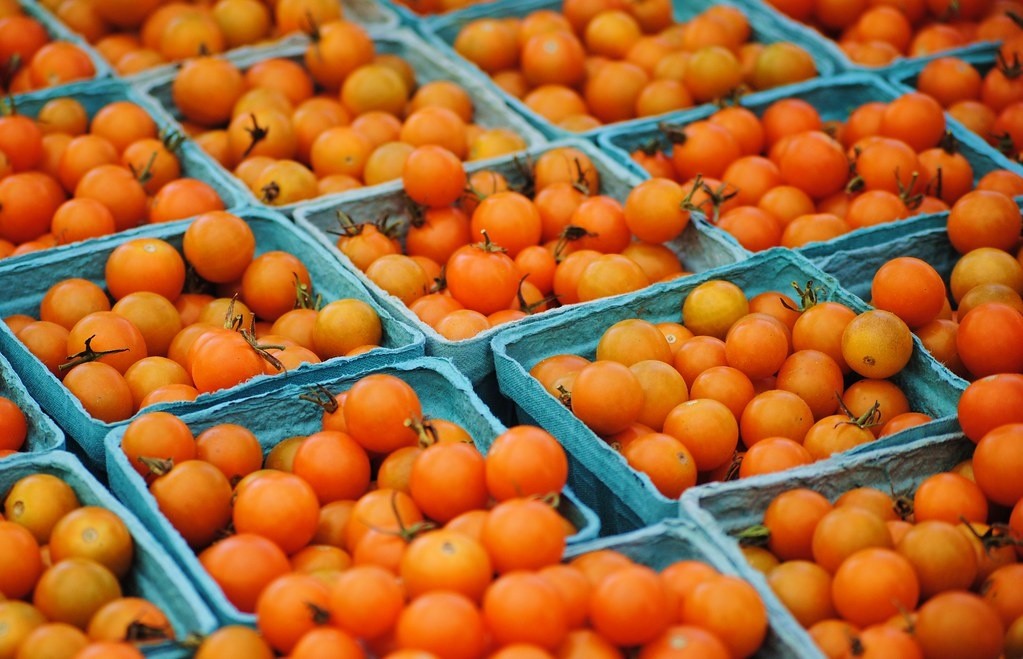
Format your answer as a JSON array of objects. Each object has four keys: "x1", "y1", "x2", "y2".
[{"x1": 0, "y1": 0, "x2": 1023, "y2": 658}]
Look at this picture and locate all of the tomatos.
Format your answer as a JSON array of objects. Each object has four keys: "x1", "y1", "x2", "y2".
[{"x1": 0, "y1": 0, "x2": 1023, "y2": 659}]
